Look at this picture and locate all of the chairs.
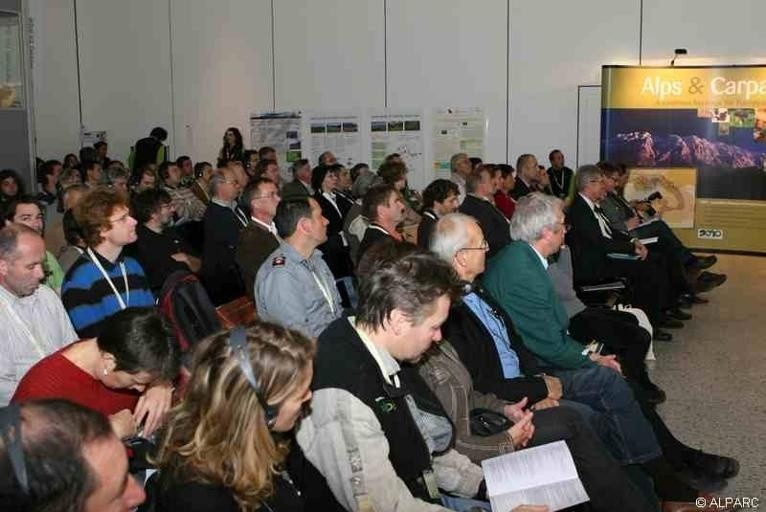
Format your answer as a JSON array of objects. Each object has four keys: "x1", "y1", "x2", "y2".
[{"x1": 215, "y1": 297, "x2": 260, "y2": 330}]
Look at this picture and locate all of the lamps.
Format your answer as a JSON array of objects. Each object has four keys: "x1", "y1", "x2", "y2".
[{"x1": 671, "y1": 49, "x2": 687, "y2": 65}]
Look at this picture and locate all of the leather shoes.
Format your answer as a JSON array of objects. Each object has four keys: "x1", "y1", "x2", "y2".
[
  {"x1": 654, "y1": 256, "x2": 726, "y2": 342},
  {"x1": 676, "y1": 449, "x2": 740, "y2": 480},
  {"x1": 660, "y1": 491, "x2": 742, "y2": 512}
]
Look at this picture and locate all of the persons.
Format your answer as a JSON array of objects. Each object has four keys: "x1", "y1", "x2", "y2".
[
  {"x1": 215, "y1": 127, "x2": 244, "y2": 164},
  {"x1": 2, "y1": 141, "x2": 742, "y2": 511},
  {"x1": 129, "y1": 127, "x2": 168, "y2": 176}
]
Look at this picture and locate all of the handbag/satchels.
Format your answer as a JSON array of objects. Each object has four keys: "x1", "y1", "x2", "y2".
[{"x1": 465, "y1": 409, "x2": 515, "y2": 435}]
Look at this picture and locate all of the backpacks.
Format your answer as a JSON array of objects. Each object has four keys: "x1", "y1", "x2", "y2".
[{"x1": 158, "y1": 271, "x2": 220, "y2": 349}]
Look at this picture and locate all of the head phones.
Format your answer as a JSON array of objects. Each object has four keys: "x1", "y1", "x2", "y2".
[
  {"x1": 231, "y1": 329, "x2": 278, "y2": 427},
  {"x1": 458, "y1": 282, "x2": 474, "y2": 296}
]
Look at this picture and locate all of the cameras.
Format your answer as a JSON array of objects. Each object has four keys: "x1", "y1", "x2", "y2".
[{"x1": 641, "y1": 190, "x2": 662, "y2": 217}]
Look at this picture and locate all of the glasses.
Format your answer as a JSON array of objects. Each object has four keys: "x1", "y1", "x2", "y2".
[{"x1": 454, "y1": 241, "x2": 490, "y2": 258}]
